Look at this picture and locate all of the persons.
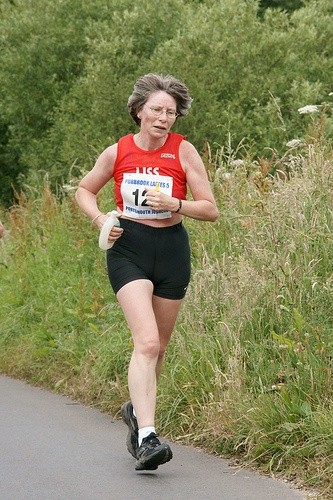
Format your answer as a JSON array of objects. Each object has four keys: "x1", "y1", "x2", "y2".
[{"x1": 75, "y1": 73, "x2": 220, "y2": 470}]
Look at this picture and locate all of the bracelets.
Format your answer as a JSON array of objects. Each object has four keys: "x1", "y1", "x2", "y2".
[
  {"x1": 175, "y1": 198, "x2": 182, "y2": 213},
  {"x1": 92, "y1": 213, "x2": 101, "y2": 224}
]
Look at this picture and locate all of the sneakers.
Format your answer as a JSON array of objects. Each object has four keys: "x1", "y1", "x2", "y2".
[{"x1": 121, "y1": 401, "x2": 173, "y2": 471}]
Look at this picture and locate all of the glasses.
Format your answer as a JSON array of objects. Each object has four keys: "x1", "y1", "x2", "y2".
[{"x1": 145, "y1": 103, "x2": 179, "y2": 119}]
